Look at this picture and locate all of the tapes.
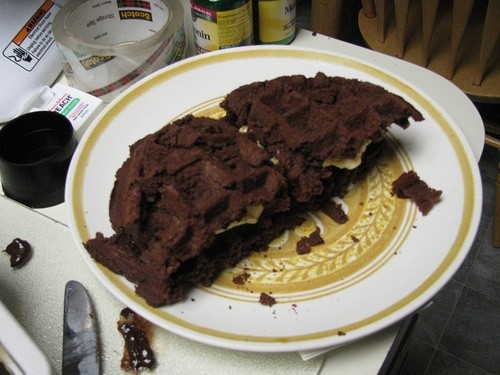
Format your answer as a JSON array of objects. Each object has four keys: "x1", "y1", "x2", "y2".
[{"x1": 52, "y1": 0, "x2": 188, "y2": 103}]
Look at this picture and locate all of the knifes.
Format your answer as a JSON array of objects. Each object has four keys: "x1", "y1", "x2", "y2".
[{"x1": 61, "y1": 280, "x2": 100, "y2": 375}]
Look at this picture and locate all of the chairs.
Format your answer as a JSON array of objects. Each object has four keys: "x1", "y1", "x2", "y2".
[{"x1": 351, "y1": 0, "x2": 499, "y2": 154}]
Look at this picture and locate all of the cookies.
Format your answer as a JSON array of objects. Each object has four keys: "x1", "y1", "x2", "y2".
[{"x1": 81, "y1": 72, "x2": 445, "y2": 309}]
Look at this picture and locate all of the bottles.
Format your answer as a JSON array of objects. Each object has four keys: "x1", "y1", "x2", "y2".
[
  {"x1": 252, "y1": 0, "x2": 296, "y2": 45},
  {"x1": 190, "y1": 0, "x2": 255, "y2": 55}
]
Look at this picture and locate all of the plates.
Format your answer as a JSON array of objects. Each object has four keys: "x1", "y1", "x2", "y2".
[{"x1": 64, "y1": 45, "x2": 483, "y2": 353}]
